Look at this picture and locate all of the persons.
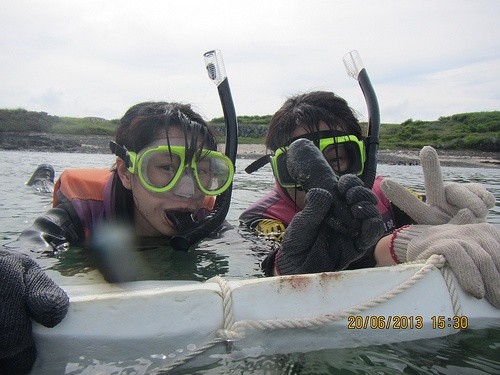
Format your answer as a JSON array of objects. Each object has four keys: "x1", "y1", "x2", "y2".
[
  {"x1": 238, "y1": 91, "x2": 500, "y2": 310},
  {"x1": 0, "y1": 102, "x2": 234, "y2": 375}
]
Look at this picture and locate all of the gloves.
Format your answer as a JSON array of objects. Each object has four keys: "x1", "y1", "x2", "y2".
[
  {"x1": 389, "y1": 208, "x2": 500, "y2": 310},
  {"x1": 379, "y1": 146, "x2": 496, "y2": 225},
  {"x1": 274, "y1": 174, "x2": 388, "y2": 276},
  {"x1": 0, "y1": 248, "x2": 69, "y2": 375}
]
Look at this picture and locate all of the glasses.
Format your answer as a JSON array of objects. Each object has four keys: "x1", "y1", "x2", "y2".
[
  {"x1": 269, "y1": 129, "x2": 366, "y2": 188},
  {"x1": 110, "y1": 140, "x2": 234, "y2": 195}
]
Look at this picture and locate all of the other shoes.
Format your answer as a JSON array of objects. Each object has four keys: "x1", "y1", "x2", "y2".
[{"x1": 26, "y1": 163, "x2": 56, "y2": 193}]
